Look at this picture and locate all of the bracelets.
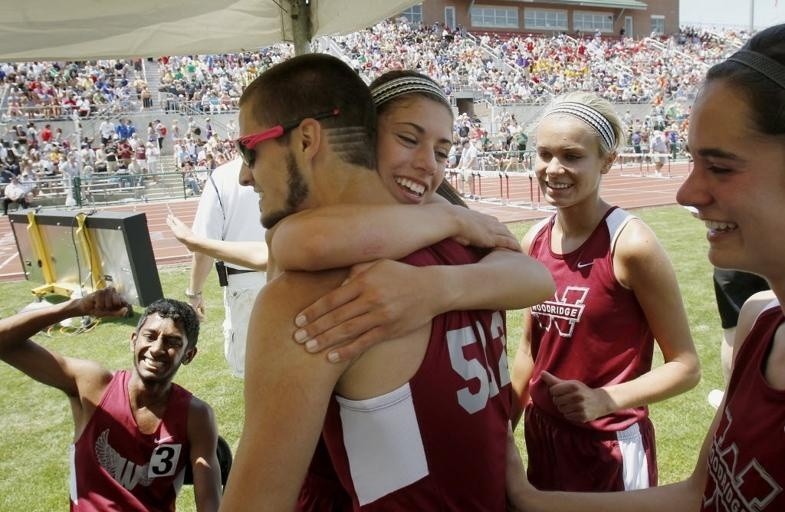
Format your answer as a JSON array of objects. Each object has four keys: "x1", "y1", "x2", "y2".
[{"x1": 184, "y1": 290, "x2": 202, "y2": 298}]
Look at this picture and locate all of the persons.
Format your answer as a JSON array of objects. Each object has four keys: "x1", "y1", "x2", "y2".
[
  {"x1": 505, "y1": 24, "x2": 785, "y2": 512},
  {"x1": 166, "y1": 216, "x2": 268, "y2": 270},
  {"x1": 708, "y1": 266, "x2": 769, "y2": 412},
  {"x1": 265, "y1": 71, "x2": 554, "y2": 365},
  {"x1": 1, "y1": 287, "x2": 222, "y2": 512},
  {"x1": 512, "y1": 92, "x2": 700, "y2": 493},
  {"x1": 187, "y1": 159, "x2": 269, "y2": 380},
  {"x1": 220, "y1": 54, "x2": 514, "y2": 512}
]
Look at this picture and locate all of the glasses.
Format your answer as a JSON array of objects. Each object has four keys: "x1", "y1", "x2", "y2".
[{"x1": 229, "y1": 104, "x2": 343, "y2": 170}]
